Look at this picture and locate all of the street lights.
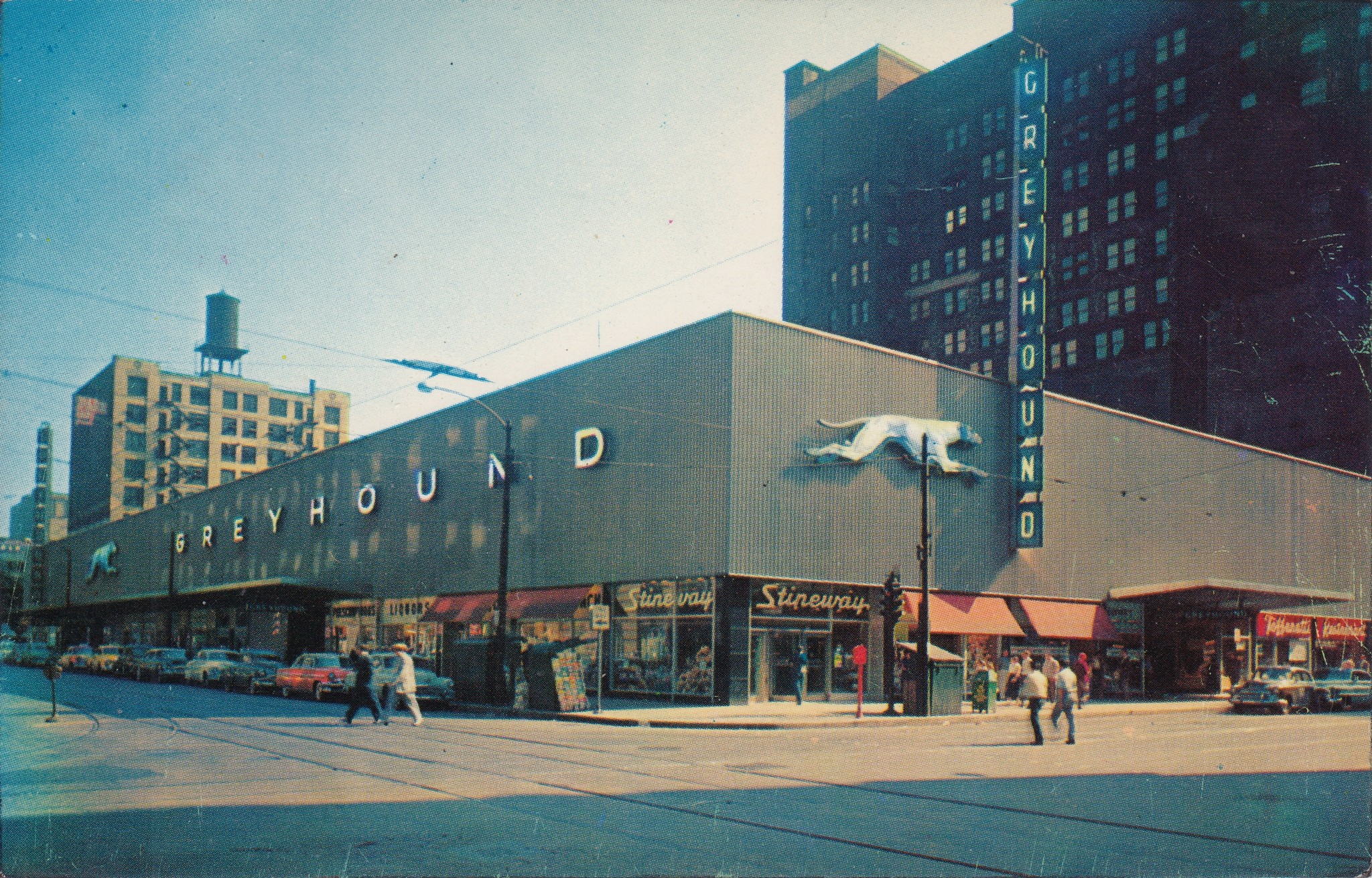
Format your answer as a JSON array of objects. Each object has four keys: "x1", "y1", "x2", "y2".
[{"x1": 418, "y1": 379, "x2": 519, "y2": 707}]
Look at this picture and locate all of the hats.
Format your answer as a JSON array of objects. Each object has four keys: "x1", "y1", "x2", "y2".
[{"x1": 392, "y1": 644, "x2": 409, "y2": 652}]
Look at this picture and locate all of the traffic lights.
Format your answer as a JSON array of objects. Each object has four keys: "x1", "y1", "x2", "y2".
[{"x1": 878, "y1": 584, "x2": 906, "y2": 622}]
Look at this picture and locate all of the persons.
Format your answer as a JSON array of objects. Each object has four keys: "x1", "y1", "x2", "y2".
[
  {"x1": 382, "y1": 642, "x2": 424, "y2": 726},
  {"x1": 338, "y1": 643, "x2": 384, "y2": 723},
  {"x1": 791, "y1": 645, "x2": 809, "y2": 705},
  {"x1": 975, "y1": 645, "x2": 1372, "y2": 748}
]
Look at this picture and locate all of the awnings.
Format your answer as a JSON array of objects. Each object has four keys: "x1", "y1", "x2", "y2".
[{"x1": 901, "y1": 587, "x2": 1120, "y2": 642}]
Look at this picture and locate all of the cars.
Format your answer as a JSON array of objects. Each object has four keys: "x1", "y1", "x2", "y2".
[
  {"x1": 1232, "y1": 665, "x2": 1315, "y2": 711},
  {"x1": 1313, "y1": 666, "x2": 1371, "y2": 710},
  {"x1": 0, "y1": 622, "x2": 455, "y2": 709}
]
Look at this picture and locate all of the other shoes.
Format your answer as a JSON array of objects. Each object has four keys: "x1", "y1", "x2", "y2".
[
  {"x1": 1066, "y1": 739, "x2": 1075, "y2": 744},
  {"x1": 1031, "y1": 741, "x2": 1043, "y2": 745},
  {"x1": 411, "y1": 721, "x2": 422, "y2": 726}
]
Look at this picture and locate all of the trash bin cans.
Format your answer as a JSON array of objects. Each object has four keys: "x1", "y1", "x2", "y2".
[
  {"x1": 521, "y1": 641, "x2": 590, "y2": 712},
  {"x1": 971, "y1": 669, "x2": 999, "y2": 714},
  {"x1": 895, "y1": 642, "x2": 965, "y2": 716},
  {"x1": 449, "y1": 633, "x2": 528, "y2": 708}
]
